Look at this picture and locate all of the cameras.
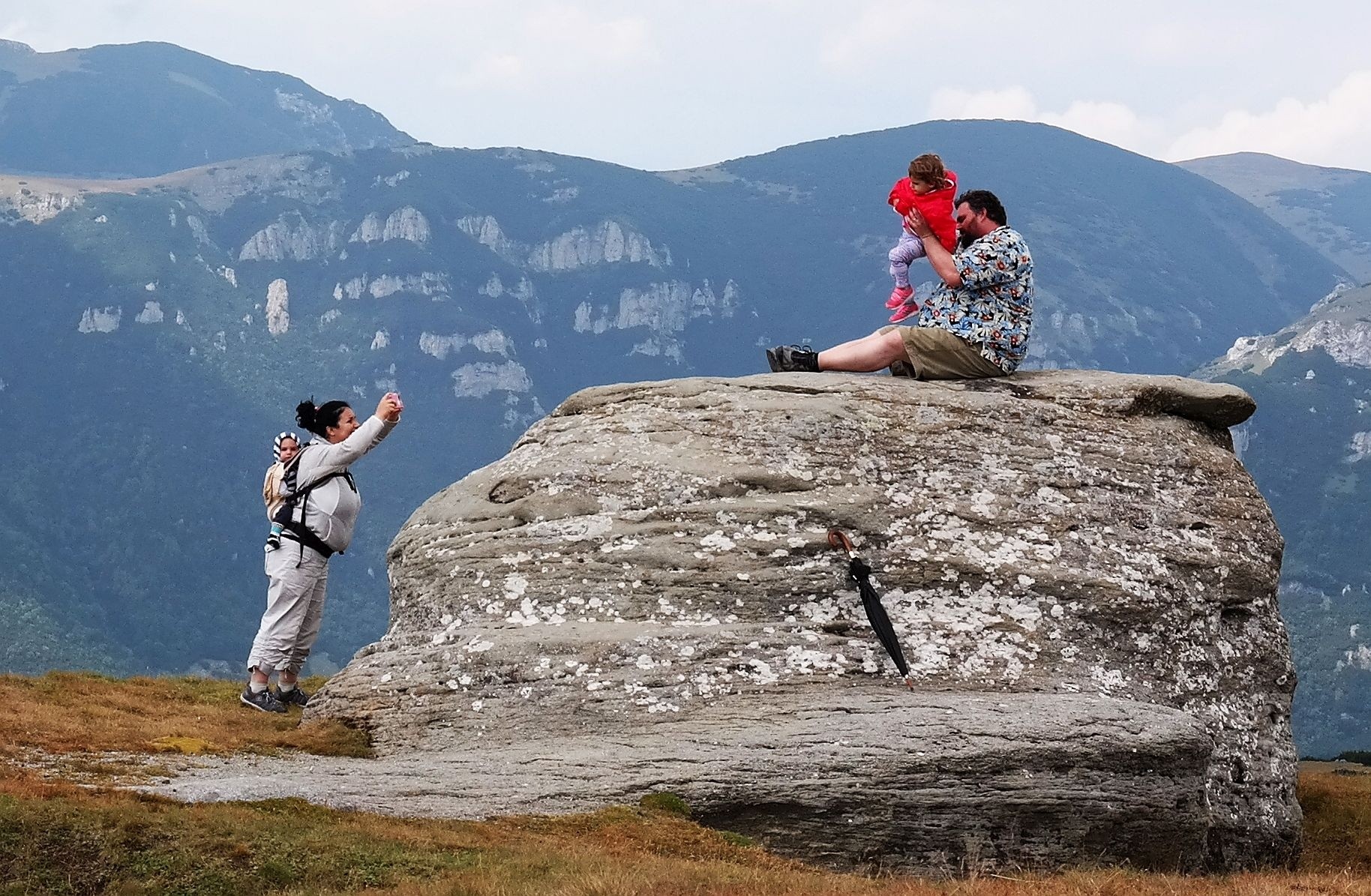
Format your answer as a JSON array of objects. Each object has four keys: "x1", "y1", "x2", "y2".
[{"x1": 388, "y1": 393, "x2": 404, "y2": 409}]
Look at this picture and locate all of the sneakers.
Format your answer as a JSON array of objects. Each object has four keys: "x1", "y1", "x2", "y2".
[
  {"x1": 888, "y1": 301, "x2": 919, "y2": 323},
  {"x1": 766, "y1": 345, "x2": 815, "y2": 373},
  {"x1": 885, "y1": 284, "x2": 915, "y2": 310},
  {"x1": 239, "y1": 682, "x2": 287, "y2": 714},
  {"x1": 273, "y1": 681, "x2": 310, "y2": 708}
]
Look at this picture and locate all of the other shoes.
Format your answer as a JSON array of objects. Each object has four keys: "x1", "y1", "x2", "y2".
[{"x1": 267, "y1": 533, "x2": 281, "y2": 548}]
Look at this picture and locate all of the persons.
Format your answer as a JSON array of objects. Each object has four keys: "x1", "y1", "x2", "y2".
[
  {"x1": 884, "y1": 154, "x2": 960, "y2": 324},
  {"x1": 241, "y1": 392, "x2": 404, "y2": 714},
  {"x1": 766, "y1": 191, "x2": 1035, "y2": 378}
]
